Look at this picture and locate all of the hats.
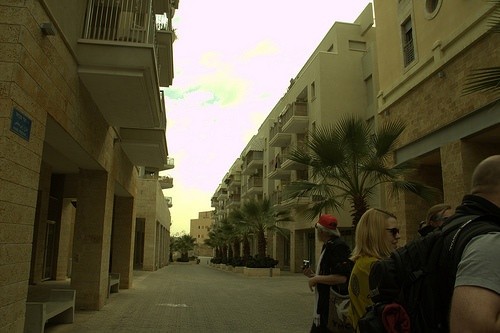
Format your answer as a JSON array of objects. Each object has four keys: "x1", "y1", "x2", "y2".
[{"x1": 316, "y1": 214, "x2": 341, "y2": 237}]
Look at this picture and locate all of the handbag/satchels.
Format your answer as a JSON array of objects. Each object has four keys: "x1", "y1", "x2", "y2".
[{"x1": 326, "y1": 287, "x2": 354, "y2": 333}]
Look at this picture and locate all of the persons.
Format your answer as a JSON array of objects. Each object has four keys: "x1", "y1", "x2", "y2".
[
  {"x1": 403, "y1": 154, "x2": 500, "y2": 333},
  {"x1": 303, "y1": 213, "x2": 354, "y2": 333},
  {"x1": 426, "y1": 204, "x2": 454, "y2": 226},
  {"x1": 348, "y1": 208, "x2": 400, "y2": 333}
]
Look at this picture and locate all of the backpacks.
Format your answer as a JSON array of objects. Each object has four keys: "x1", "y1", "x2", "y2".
[{"x1": 355, "y1": 215, "x2": 500, "y2": 333}]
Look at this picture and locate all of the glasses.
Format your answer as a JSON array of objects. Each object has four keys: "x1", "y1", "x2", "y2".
[{"x1": 385, "y1": 228, "x2": 399, "y2": 235}]
